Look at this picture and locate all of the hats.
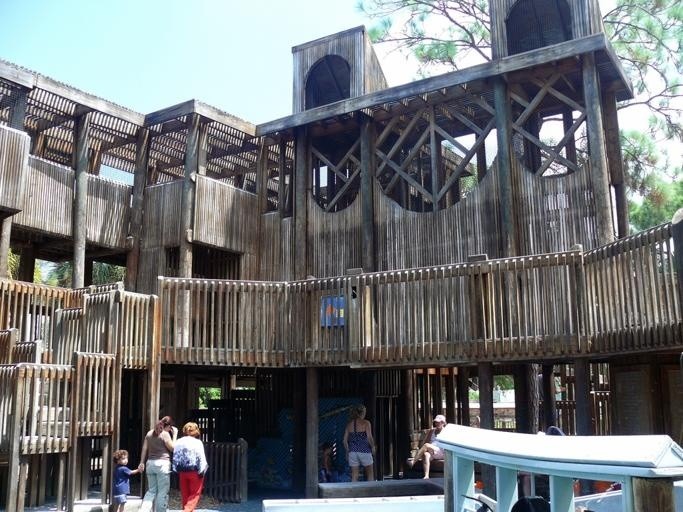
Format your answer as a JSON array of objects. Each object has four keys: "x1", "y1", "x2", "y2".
[{"x1": 434, "y1": 415, "x2": 445, "y2": 422}]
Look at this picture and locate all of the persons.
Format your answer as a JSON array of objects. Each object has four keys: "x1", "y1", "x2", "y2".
[
  {"x1": 138, "y1": 414, "x2": 178, "y2": 512},
  {"x1": 510, "y1": 494, "x2": 550, "y2": 512},
  {"x1": 516, "y1": 468, "x2": 548, "y2": 498},
  {"x1": 319, "y1": 442, "x2": 332, "y2": 482},
  {"x1": 342, "y1": 403, "x2": 377, "y2": 483},
  {"x1": 405, "y1": 414, "x2": 447, "y2": 480},
  {"x1": 535, "y1": 364, "x2": 567, "y2": 433},
  {"x1": 109, "y1": 449, "x2": 145, "y2": 512},
  {"x1": 171, "y1": 421, "x2": 208, "y2": 512}
]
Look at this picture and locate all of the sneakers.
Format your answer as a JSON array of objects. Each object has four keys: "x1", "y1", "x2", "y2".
[{"x1": 408, "y1": 460, "x2": 413, "y2": 467}]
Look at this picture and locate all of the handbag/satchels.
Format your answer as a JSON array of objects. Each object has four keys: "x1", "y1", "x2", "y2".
[{"x1": 174, "y1": 449, "x2": 199, "y2": 471}]
